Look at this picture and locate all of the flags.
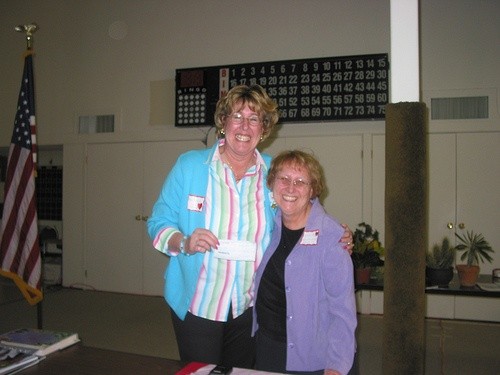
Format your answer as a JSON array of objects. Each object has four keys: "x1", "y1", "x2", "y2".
[{"x1": 0, "y1": 49, "x2": 42, "y2": 306}]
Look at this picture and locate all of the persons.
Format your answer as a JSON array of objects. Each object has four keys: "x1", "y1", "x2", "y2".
[
  {"x1": 250, "y1": 148, "x2": 358, "y2": 375},
  {"x1": 147, "y1": 84, "x2": 353, "y2": 367}
]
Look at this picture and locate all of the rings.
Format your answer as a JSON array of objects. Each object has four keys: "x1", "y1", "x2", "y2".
[{"x1": 348, "y1": 242, "x2": 354, "y2": 247}]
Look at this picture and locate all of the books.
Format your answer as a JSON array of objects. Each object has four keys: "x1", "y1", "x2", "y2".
[{"x1": 0, "y1": 327, "x2": 80, "y2": 375}]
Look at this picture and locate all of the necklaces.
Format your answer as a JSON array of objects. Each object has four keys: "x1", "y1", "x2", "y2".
[{"x1": 230, "y1": 166, "x2": 242, "y2": 182}]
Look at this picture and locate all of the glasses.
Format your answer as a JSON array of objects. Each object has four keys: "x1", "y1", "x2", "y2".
[
  {"x1": 228, "y1": 112, "x2": 262, "y2": 126},
  {"x1": 276, "y1": 175, "x2": 309, "y2": 189}
]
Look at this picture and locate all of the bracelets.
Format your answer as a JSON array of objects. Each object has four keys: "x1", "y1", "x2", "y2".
[{"x1": 179, "y1": 236, "x2": 192, "y2": 256}]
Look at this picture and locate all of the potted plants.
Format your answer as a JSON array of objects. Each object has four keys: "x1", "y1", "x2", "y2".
[
  {"x1": 425, "y1": 235, "x2": 456, "y2": 288},
  {"x1": 349, "y1": 222, "x2": 385, "y2": 283},
  {"x1": 454, "y1": 229, "x2": 495, "y2": 287}
]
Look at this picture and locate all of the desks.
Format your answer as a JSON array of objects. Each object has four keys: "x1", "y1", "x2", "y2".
[{"x1": 14, "y1": 344, "x2": 190, "y2": 375}]
